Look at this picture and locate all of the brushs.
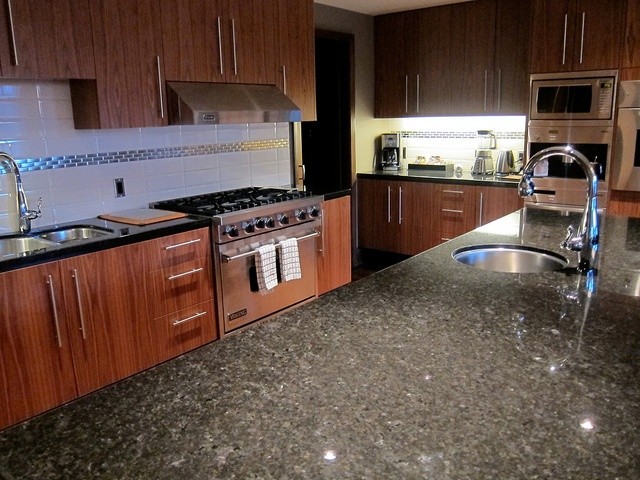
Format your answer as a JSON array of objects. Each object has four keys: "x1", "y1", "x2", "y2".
[{"x1": 380, "y1": 133, "x2": 400, "y2": 171}]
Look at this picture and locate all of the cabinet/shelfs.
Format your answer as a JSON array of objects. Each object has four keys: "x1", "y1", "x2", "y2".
[{"x1": 399, "y1": 146, "x2": 408, "y2": 176}]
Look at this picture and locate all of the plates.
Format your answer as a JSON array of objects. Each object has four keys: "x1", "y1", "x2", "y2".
[
  {"x1": 357, "y1": 179, "x2": 432, "y2": 256},
  {"x1": 317, "y1": 194, "x2": 354, "y2": 298},
  {"x1": 607, "y1": 190, "x2": 640, "y2": 217},
  {"x1": 0, "y1": 227, "x2": 147, "y2": 436},
  {"x1": 139, "y1": 227, "x2": 218, "y2": 374},
  {"x1": 373, "y1": 0, "x2": 452, "y2": 118},
  {"x1": 433, "y1": 183, "x2": 476, "y2": 247},
  {"x1": 167, "y1": 1, "x2": 319, "y2": 122},
  {"x1": 618, "y1": 0, "x2": 640, "y2": 81},
  {"x1": 69, "y1": 1, "x2": 167, "y2": 130},
  {"x1": 0, "y1": 1, "x2": 96, "y2": 80},
  {"x1": 528, "y1": 0, "x2": 622, "y2": 75},
  {"x1": 476, "y1": 186, "x2": 524, "y2": 228},
  {"x1": 450, "y1": 0, "x2": 537, "y2": 117}
]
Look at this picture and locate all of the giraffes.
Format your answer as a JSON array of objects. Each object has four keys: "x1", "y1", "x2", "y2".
[{"x1": 148, "y1": 186, "x2": 324, "y2": 226}]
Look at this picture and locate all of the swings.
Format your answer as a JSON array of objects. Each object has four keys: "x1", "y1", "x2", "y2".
[{"x1": 470, "y1": 130, "x2": 497, "y2": 175}]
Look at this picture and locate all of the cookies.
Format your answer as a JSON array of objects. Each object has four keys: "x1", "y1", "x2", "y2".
[{"x1": 530, "y1": 78, "x2": 615, "y2": 120}]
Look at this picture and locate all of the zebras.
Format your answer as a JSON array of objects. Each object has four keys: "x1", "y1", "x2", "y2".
[{"x1": 212, "y1": 195, "x2": 324, "y2": 341}]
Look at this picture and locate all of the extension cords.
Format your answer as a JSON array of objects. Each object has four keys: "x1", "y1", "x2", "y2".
[{"x1": 493, "y1": 150, "x2": 515, "y2": 179}]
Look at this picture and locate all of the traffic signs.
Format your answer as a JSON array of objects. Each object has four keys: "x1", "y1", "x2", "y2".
[{"x1": 517, "y1": 150, "x2": 523, "y2": 165}]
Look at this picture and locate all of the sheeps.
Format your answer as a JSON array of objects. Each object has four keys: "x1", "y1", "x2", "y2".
[
  {"x1": 517, "y1": 147, "x2": 600, "y2": 275},
  {"x1": 0, "y1": 151, "x2": 43, "y2": 234}
]
